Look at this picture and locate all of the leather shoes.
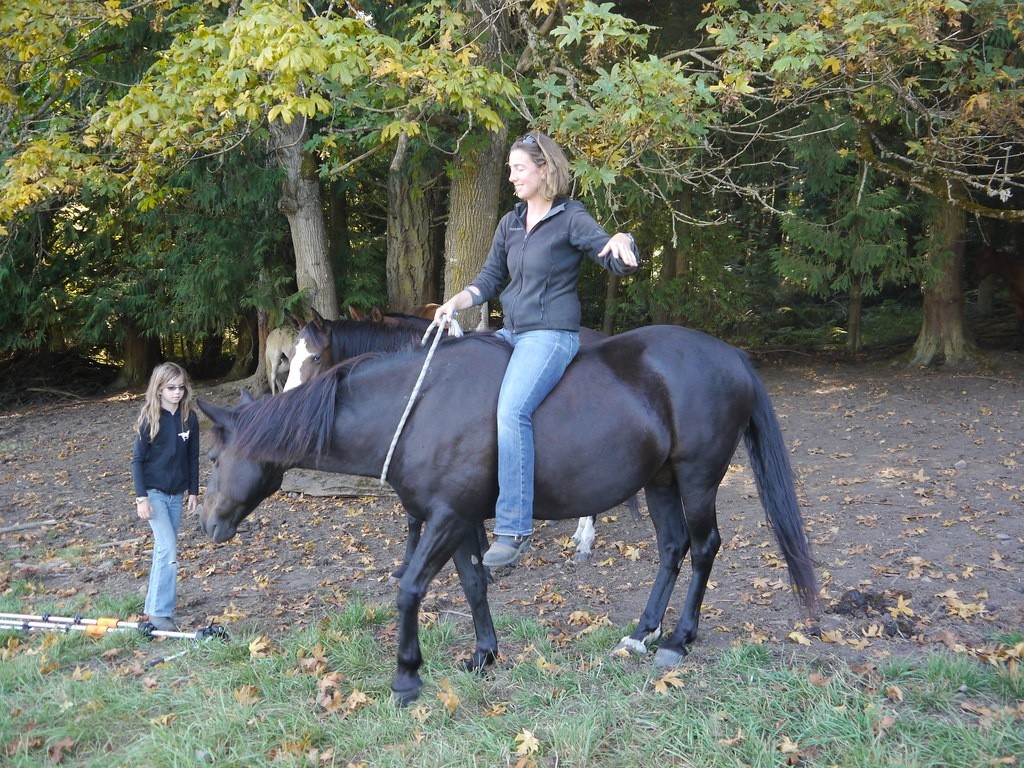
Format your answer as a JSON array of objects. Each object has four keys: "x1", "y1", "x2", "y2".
[{"x1": 481, "y1": 532, "x2": 529, "y2": 566}]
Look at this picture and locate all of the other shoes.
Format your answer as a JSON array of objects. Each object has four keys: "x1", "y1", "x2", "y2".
[{"x1": 147, "y1": 615, "x2": 176, "y2": 632}]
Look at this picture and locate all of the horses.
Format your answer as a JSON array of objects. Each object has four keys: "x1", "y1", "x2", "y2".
[{"x1": 202, "y1": 304, "x2": 814, "y2": 709}]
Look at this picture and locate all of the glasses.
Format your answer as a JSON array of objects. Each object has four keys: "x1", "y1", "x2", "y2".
[
  {"x1": 164, "y1": 383, "x2": 186, "y2": 391},
  {"x1": 517, "y1": 135, "x2": 545, "y2": 158}
]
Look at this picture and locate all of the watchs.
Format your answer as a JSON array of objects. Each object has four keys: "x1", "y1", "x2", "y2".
[{"x1": 136, "y1": 497, "x2": 147, "y2": 503}]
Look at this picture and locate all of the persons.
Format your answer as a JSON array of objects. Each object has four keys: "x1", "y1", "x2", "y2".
[
  {"x1": 426, "y1": 130, "x2": 641, "y2": 566},
  {"x1": 130, "y1": 363, "x2": 200, "y2": 631}
]
